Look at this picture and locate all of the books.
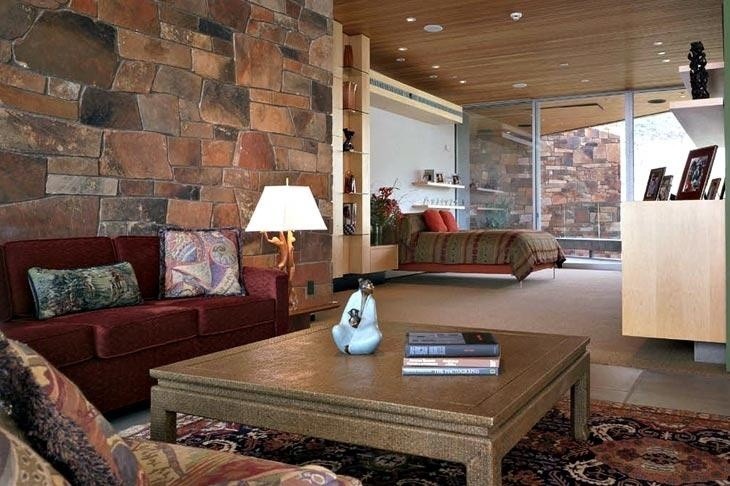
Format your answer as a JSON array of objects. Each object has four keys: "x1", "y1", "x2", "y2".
[{"x1": 400, "y1": 329, "x2": 503, "y2": 376}]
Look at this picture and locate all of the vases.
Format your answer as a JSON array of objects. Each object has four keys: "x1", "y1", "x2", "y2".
[{"x1": 373, "y1": 223, "x2": 384, "y2": 246}]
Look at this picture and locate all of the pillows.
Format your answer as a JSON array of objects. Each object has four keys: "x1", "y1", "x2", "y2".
[
  {"x1": 26, "y1": 261, "x2": 145, "y2": 320},
  {"x1": 157, "y1": 226, "x2": 248, "y2": 300},
  {"x1": 0, "y1": 330, "x2": 151, "y2": 486},
  {"x1": 438, "y1": 209, "x2": 460, "y2": 232},
  {"x1": 421, "y1": 208, "x2": 448, "y2": 233}
]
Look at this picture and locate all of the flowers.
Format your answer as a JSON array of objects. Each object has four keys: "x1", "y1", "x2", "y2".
[{"x1": 370, "y1": 175, "x2": 404, "y2": 230}]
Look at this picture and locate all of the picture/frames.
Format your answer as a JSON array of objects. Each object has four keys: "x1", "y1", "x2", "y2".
[
  {"x1": 657, "y1": 175, "x2": 674, "y2": 201},
  {"x1": 643, "y1": 166, "x2": 667, "y2": 201},
  {"x1": 675, "y1": 145, "x2": 719, "y2": 200},
  {"x1": 706, "y1": 178, "x2": 722, "y2": 200}
]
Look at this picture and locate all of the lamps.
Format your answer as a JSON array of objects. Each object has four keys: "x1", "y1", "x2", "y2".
[{"x1": 244, "y1": 177, "x2": 328, "y2": 311}]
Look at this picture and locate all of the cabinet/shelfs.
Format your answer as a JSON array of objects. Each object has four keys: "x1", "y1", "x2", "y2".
[
  {"x1": 668, "y1": 61, "x2": 725, "y2": 148},
  {"x1": 410, "y1": 181, "x2": 467, "y2": 210},
  {"x1": 342, "y1": 66, "x2": 370, "y2": 236},
  {"x1": 620, "y1": 201, "x2": 728, "y2": 344},
  {"x1": 472, "y1": 187, "x2": 506, "y2": 211}
]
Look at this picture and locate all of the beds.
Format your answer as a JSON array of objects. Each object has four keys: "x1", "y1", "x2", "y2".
[{"x1": 384, "y1": 212, "x2": 566, "y2": 289}]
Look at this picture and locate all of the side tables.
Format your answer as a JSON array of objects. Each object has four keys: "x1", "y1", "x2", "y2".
[{"x1": 288, "y1": 301, "x2": 342, "y2": 331}]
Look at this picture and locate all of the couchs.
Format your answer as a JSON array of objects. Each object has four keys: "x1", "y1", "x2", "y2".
[
  {"x1": 0, "y1": 232, "x2": 290, "y2": 415},
  {"x1": 0, "y1": 398, "x2": 363, "y2": 486}
]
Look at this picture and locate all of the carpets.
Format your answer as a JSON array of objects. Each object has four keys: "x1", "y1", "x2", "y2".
[{"x1": 121, "y1": 393, "x2": 730, "y2": 486}]
